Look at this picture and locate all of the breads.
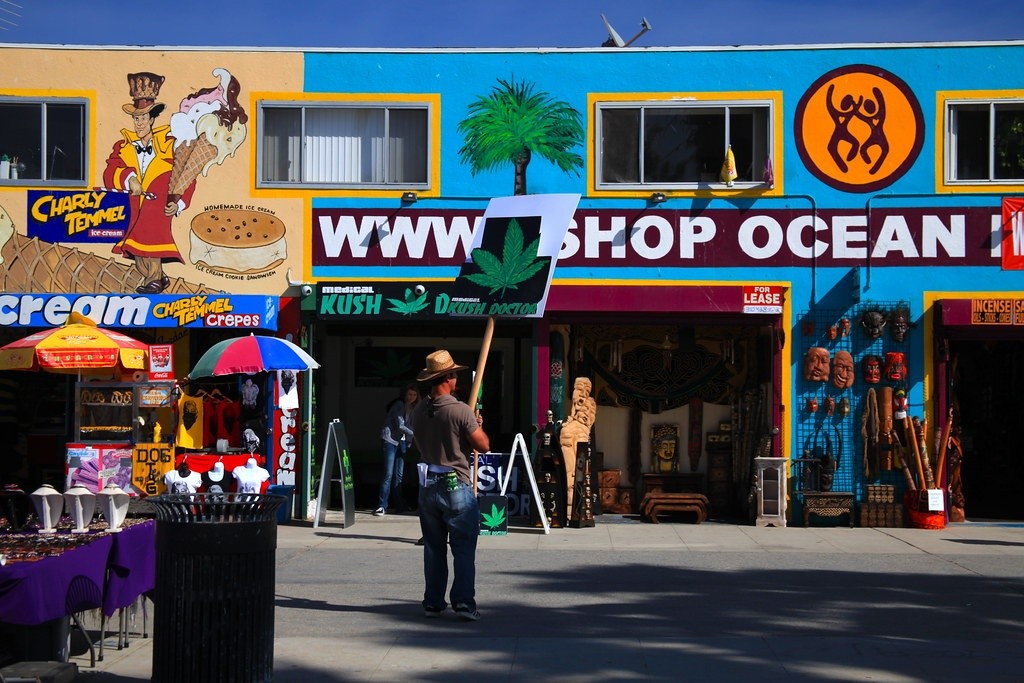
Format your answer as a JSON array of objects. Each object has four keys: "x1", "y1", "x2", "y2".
[{"x1": 81, "y1": 389, "x2": 133, "y2": 404}]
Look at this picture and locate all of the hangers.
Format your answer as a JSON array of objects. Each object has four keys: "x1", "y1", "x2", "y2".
[{"x1": 176, "y1": 385, "x2": 234, "y2": 404}]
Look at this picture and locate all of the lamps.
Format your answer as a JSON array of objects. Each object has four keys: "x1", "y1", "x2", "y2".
[
  {"x1": 402, "y1": 192, "x2": 418, "y2": 202},
  {"x1": 651, "y1": 192, "x2": 667, "y2": 203}
]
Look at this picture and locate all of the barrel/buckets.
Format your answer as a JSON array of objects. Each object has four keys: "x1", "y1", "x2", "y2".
[{"x1": 907, "y1": 489, "x2": 947, "y2": 528}]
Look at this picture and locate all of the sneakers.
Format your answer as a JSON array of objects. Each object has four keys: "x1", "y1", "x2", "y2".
[
  {"x1": 451, "y1": 600, "x2": 481, "y2": 620},
  {"x1": 425, "y1": 603, "x2": 448, "y2": 615},
  {"x1": 373, "y1": 506, "x2": 387, "y2": 516},
  {"x1": 397, "y1": 506, "x2": 417, "y2": 514}
]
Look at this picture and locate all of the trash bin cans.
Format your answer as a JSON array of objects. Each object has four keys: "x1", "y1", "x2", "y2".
[{"x1": 143, "y1": 493, "x2": 289, "y2": 683}]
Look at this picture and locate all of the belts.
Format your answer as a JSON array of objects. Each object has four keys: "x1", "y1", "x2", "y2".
[{"x1": 427, "y1": 472, "x2": 446, "y2": 479}]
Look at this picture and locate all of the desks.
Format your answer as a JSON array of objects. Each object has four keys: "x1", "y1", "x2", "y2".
[
  {"x1": 0, "y1": 516, "x2": 157, "y2": 668},
  {"x1": 175, "y1": 452, "x2": 272, "y2": 515},
  {"x1": 801, "y1": 491, "x2": 856, "y2": 528}
]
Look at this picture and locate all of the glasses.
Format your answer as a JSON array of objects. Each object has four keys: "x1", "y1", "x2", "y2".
[{"x1": 427, "y1": 398, "x2": 435, "y2": 417}]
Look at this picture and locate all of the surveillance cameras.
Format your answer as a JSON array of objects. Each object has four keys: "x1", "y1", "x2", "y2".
[{"x1": 302, "y1": 286, "x2": 312, "y2": 296}]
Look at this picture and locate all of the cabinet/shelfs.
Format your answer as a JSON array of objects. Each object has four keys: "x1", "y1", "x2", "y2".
[
  {"x1": 705, "y1": 440, "x2": 734, "y2": 517},
  {"x1": 642, "y1": 473, "x2": 706, "y2": 493},
  {"x1": 754, "y1": 457, "x2": 788, "y2": 528},
  {"x1": 596, "y1": 451, "x2": 639, "y2": 514}
]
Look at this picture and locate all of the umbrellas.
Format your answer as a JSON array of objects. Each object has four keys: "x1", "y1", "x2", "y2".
[
  {"x1": 0, "y1": 321, "x2": 147, "y2": 381},
  {"x1": 186, "y1": 333, "x2": 321, "y2": 446}
]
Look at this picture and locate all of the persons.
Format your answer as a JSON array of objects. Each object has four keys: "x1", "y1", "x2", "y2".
[
  {"x1": 418, "y1": 351, "x2": 490, "y2": 619},
  {"x1": 243, "y1": 379, "x2": 257, "y2": 402},
  {"x1": 375, "y1": 387, "x2": 419, "y2": 515},
  {"x1": 163, "y1": 457, "x2": 269, "y2": 503},
  {"x1": 560, "y1": 379, "x2": 596, "y2": 514}
]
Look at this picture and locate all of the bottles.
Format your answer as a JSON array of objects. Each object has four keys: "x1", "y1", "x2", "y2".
[
  {"x1": 11, "y1": 163, "x2": 18, "y2": 180},
  {"x1": 447, "y1": 471, "x2": 462, "y2": 510},
  {"x1": 0, "y1": 153, "x2": 10, "y2": 179}
]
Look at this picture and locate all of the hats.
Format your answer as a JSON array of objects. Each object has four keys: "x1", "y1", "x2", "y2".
[{"x1": 416, "y1": 349, "x2": 470, "y2": 381}]
